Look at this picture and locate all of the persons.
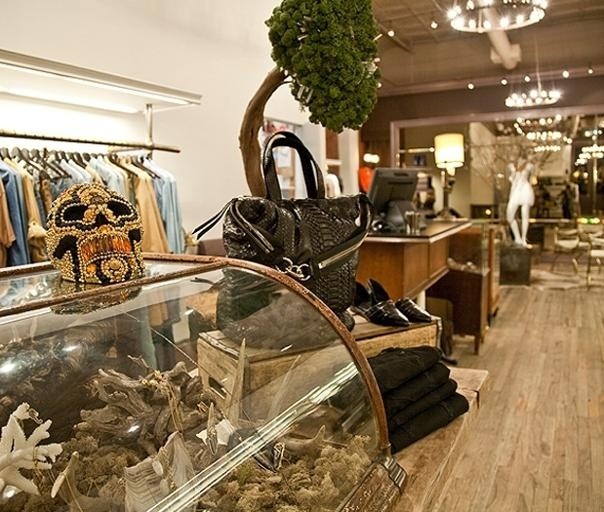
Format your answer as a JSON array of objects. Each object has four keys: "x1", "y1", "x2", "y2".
[{"x1": 507, "y1": 148, "x2": 535, "y2": 246}]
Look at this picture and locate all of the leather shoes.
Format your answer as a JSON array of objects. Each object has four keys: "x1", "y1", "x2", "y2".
[
  {"x1": 367, "y1": 279, "x2": 432, "y2": 323},
  {"x1": 347, "y1": 280, "x2": 408, "y2": 326}
]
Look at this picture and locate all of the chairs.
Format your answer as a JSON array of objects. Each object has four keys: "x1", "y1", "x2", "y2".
[{"x1": 549, "y1": 224, "x2": 604, "y2": 291}]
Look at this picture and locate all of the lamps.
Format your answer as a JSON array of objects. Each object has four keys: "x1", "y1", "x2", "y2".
[
  {"x1": 426, "y1": 133, "x2": 463, "y2": 223},
  {"x1": 445, "y1": 0, "x2": 561, "y2": 108}
]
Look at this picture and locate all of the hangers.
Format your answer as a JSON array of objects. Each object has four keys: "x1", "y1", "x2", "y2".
[{"x1": 0, "y1": 131, "x2": 161, "y2": 185}]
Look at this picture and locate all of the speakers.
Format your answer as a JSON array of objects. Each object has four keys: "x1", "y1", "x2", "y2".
[{"x1": 497, "y1": 242, "x2": 532, "y2": 286}]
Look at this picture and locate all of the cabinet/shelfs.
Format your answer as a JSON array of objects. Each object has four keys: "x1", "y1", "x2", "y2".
[{"x1": 1, "y1": 251, "x2": 405, "y2": 511}]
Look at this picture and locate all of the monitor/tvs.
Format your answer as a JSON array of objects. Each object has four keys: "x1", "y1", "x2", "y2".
[{"x1": 367, "y1": 167, "x2": 420, "y2": 216}]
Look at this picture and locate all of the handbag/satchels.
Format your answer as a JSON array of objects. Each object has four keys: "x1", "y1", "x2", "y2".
[{"x1": 215, "y1": 131, "x2": 371, "y2": 351}]
